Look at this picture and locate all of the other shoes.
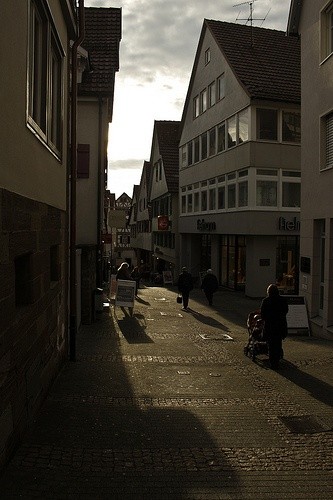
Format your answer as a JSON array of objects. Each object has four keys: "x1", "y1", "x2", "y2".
[
  {"x1": 207, "y1": 304, "x2": 212, "y2": 306},
  {"x1": 182, "y1": 306, "x2": 187, "y2": 309}
]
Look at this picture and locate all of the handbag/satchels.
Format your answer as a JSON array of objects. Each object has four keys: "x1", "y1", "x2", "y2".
[{"x1": 177, "y1": 293, "x2": 183, "y2": 304}]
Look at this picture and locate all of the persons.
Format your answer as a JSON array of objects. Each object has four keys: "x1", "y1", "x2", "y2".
[
  {"x1": 260, "y1": 283, "x2": 289, "y2": 370},
  {"x1": 177, "y1": 267, "x2": 194, "y2": 310},
  {"x1": 200, "y1": 269, "x2": 218, "y2": 306},
  {"x1": 253, "y1": 313, "x2": 261, "y2": 325},
  {"x1": 115, "y1": 262, "x2": 131, "y2": 281},
  {"x1": 130, "y1": 266, "x2": 141, "y2": 297}
]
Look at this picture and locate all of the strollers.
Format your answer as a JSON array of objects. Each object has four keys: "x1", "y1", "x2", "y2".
[{"x1": 244, "y1": 312, "x2": 269, "y2": 360}]
